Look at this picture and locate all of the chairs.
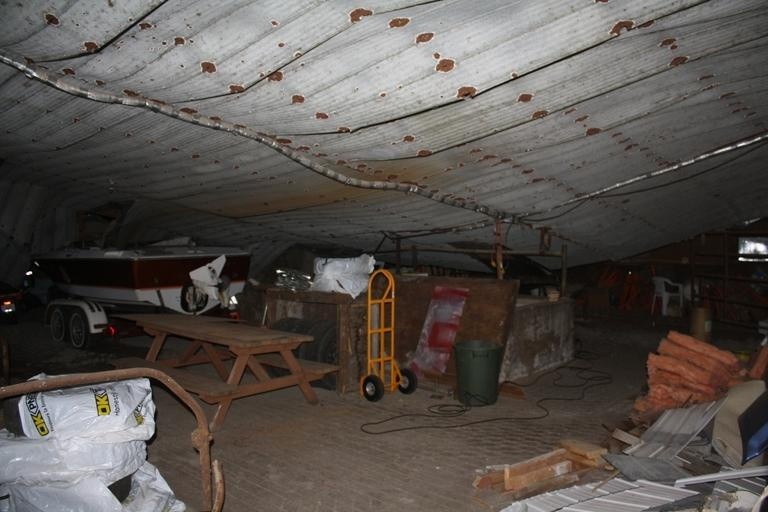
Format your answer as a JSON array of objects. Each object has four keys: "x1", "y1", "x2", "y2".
[{"x1": 649, "y1": 276, "x2": 684, "y2": 317}]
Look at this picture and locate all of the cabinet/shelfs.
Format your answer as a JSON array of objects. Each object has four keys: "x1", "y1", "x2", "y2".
[{"x1": 259, "y1": 281, "x2": 382, "y2": 398}]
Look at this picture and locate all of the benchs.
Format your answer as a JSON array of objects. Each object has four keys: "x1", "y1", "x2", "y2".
[{"x1": 107, "y1": 350, "x2": 344, "y2": 431}]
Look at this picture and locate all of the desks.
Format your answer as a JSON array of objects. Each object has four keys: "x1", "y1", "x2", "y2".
[{"x1": 113, "y1": 309, "x2": 319, "y2": 437}]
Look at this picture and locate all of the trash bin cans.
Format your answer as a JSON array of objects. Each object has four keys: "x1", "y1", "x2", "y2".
[{"x1": 454, "y1": 340, "x2": 503, "y2": 407}]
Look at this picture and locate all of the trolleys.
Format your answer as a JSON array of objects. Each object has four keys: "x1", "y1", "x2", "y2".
[{"x1": 358, "y1": 267, "x2": 417, "y2": 401}]
